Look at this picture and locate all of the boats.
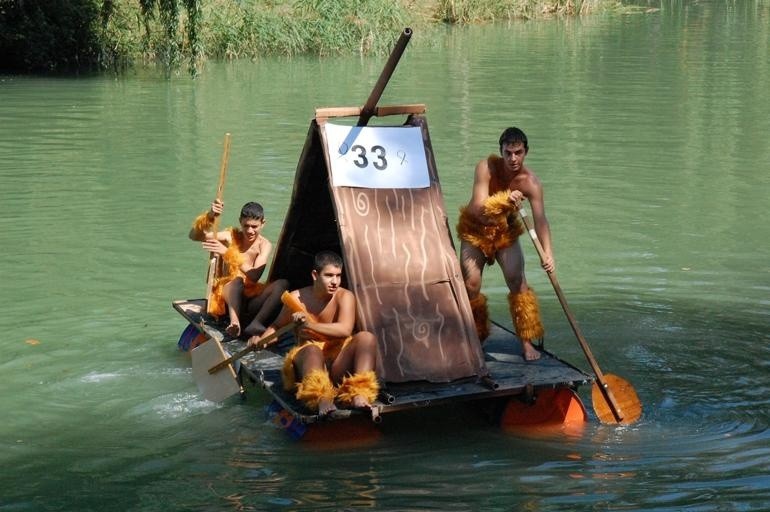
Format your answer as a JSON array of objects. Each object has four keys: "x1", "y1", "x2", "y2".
[{"x1": 171, "y1": 28, "x2": 595, "y2": 448}]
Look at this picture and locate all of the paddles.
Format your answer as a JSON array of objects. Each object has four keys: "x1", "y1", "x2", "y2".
[
  {"x1": 202, "y1": 134, "x2": 232, "y2": 312},
  {"x1": 190, "y1": 317, "x2": 304, "y2": 403},
  {"x1": 516, "y1": 204, "x2": 642, "y2": 425}
]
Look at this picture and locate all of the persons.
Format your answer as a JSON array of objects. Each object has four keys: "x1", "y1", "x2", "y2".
[
  {"x1": 187, "y1": 198, "x2": 290, "y2": 339},
  {"x1": 248, "y1": 249, "x2": 379, "y2": 418},
  {"x1": 458, "y1": 126, "x2": 555, "y2": 360}
]
